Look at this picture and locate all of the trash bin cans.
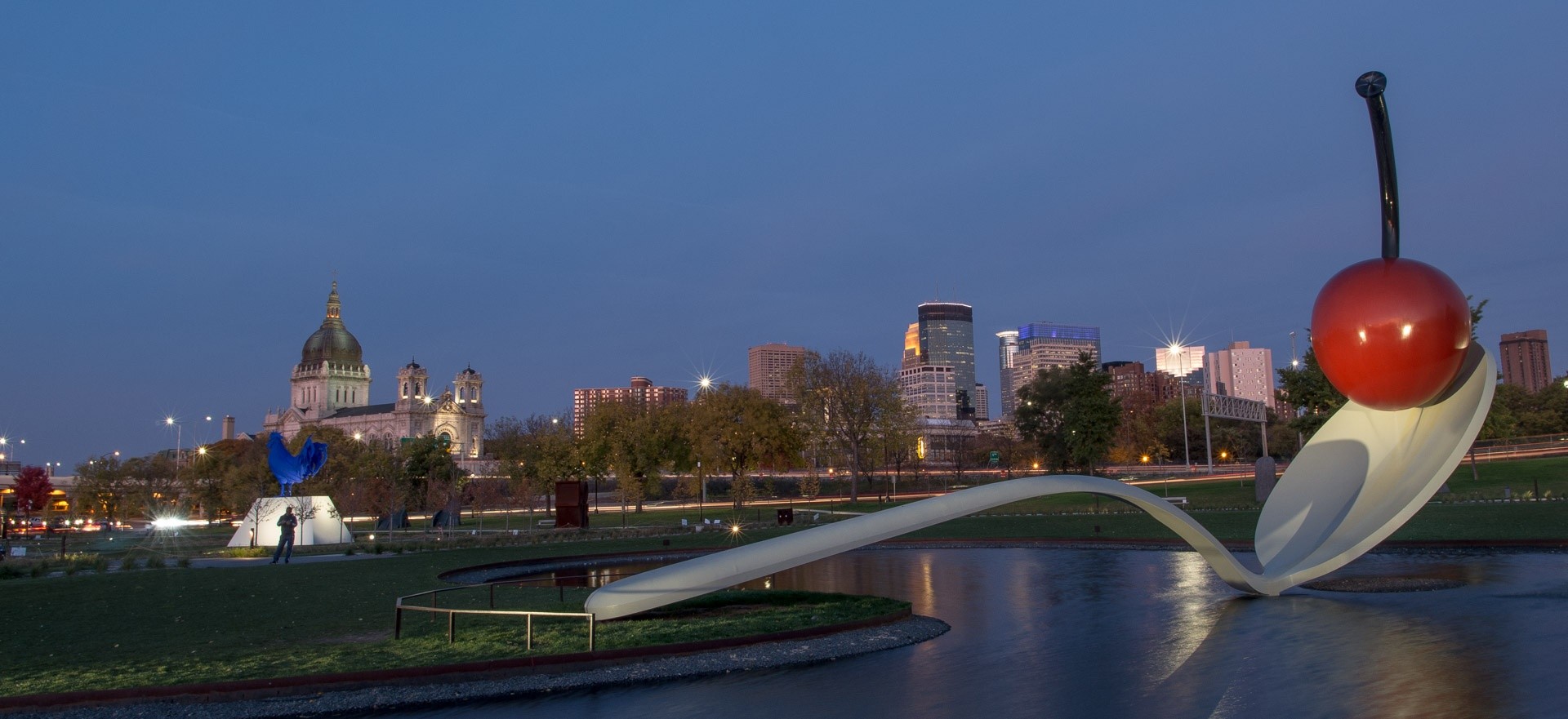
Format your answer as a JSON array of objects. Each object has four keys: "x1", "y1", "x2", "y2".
[{"x1": 776, "y1": 508, "x2": 794, "y2": 526}]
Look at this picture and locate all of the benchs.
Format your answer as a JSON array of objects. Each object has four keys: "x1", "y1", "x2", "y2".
[{"x1": 1162, "y1": 497, "x2": 1188, "y2": 509}]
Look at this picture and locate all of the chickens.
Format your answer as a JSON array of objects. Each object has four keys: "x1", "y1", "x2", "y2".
[{"x1": 266, "y1": 430, "x2": 328, "y2": 497}]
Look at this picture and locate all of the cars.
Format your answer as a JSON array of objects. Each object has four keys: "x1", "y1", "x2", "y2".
[{"x1": 0, "y1": 512, "x2": 121, "y2": 528}]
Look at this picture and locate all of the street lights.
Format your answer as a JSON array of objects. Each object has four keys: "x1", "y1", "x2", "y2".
[
  {"x1": 0, "y1": 438, "x2": 25, "y2": 461},
  {"x1": 166, "y1": 418, "x2": 181, "y2": 481},
  {"x1": 192, "y1": 416, "x2": 211, "y2": 482},
  {"x1": 46, "y1": 462, "x2": 60, "y2": 476},
  {"x1": 1169, "y1": 344, "x2": 1191, "y2": 471}
]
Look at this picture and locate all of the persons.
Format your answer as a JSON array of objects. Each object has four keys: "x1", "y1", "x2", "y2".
[{"x1": 273, "y1": 507, "x2": 297, "y2": 564}]
